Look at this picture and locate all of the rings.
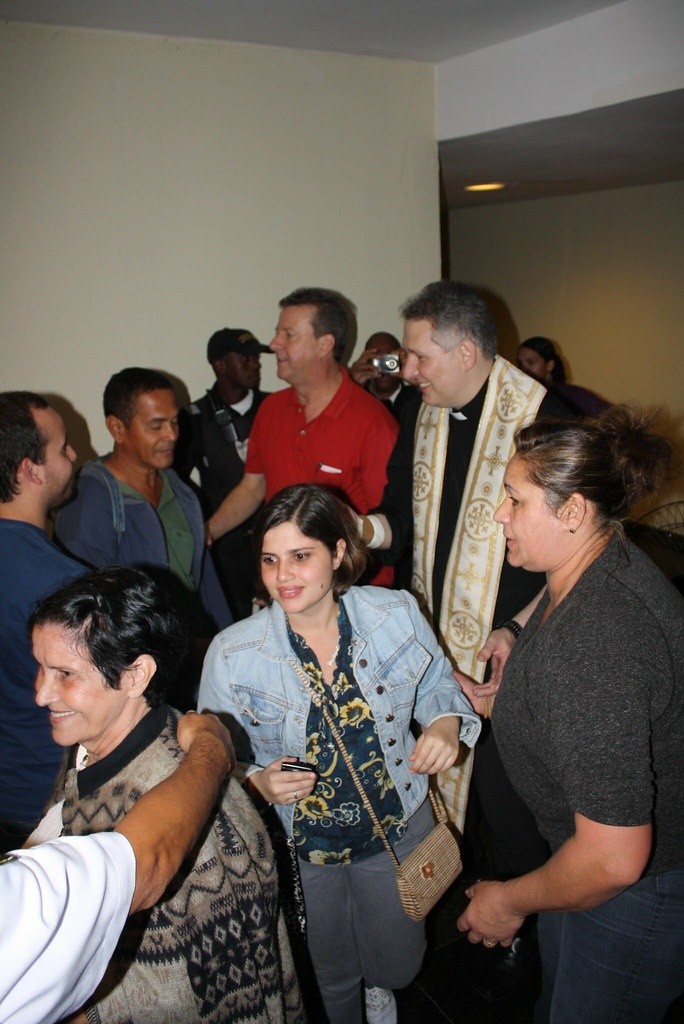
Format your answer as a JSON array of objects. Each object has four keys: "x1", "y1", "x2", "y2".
[
  {"x1": 486, "y1": 939, "x2": 496, "y2": 946},
  {"x1": 294, "y1": 791, "x2": 297, "y2": 799}
]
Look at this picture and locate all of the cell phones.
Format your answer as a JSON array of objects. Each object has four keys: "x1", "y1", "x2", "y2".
[{"x1": 281, "y1": 761, "x2": 313, "y2": 773}]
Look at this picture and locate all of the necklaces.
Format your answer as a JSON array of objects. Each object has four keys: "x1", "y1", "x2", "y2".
[{"x1": 328, "y1": 636, "x2": 341, "y2": 666}]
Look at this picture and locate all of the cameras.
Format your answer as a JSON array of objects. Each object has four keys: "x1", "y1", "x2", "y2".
[{"x1": 369, "y1": 354, "x2": 401, "y2": 374}]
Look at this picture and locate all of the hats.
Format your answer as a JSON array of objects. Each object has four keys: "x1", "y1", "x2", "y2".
[{"x1": 207, "y1": 328, "x2": 277, "y2": 363}]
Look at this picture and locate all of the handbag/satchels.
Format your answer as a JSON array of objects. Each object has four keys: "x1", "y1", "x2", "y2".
[{"x1": 396, "y1": 822, "x2": 465, "y2": 922}]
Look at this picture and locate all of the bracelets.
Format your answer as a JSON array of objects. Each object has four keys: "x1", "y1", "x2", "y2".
[{"x1": 483, "y1": 696, "x2": 492, "y2": 719}]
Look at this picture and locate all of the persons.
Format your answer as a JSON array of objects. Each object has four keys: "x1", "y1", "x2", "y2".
[{"x1": 0, "y1": 277, "x2": 684, "y2": 1024}]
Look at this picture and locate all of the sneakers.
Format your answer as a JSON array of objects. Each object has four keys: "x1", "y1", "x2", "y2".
[{"x1": 365, "y1": 986, "x2": 397, "y2": 1024}]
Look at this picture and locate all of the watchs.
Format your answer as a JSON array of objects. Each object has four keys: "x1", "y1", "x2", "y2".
[{"x1": 496, "y1": 619, "x2": 522, "y2": 638}]
